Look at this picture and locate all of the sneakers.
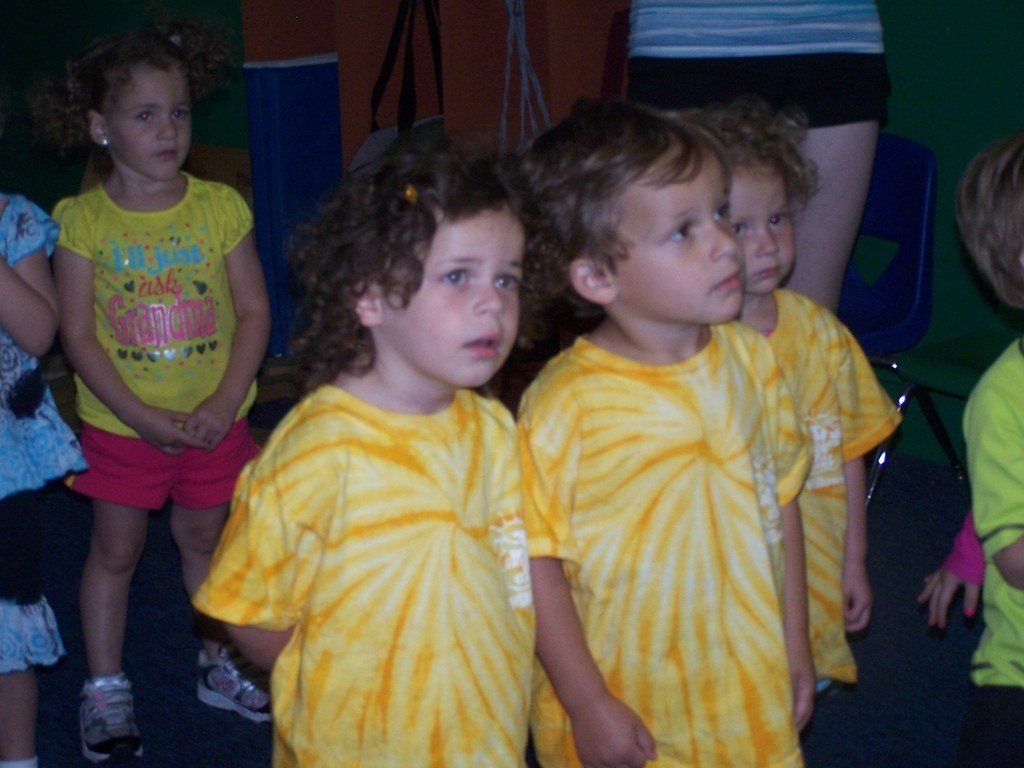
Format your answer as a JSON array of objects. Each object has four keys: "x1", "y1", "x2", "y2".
[
  {"x1": 199, "y1": 647, "x2": 273, "y2": 723},
  {"x1": 82, "y1": 675, "x2": 145, "y2": 760}
]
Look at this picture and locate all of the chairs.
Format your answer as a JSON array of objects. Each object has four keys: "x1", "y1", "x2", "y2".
[{"x1": 835, "y1": 132, "x2": 972, "y2": 512}]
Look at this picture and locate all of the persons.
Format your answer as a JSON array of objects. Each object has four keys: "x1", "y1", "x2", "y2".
[
  {"x1": 0, "y1": 191, "x2": 68, "y2": 768},
  {"x1": 189, "y1": 146, "x2": 539, "y2": 768},
  {"x1": 916, "y1": 509, "x2": 986, "y2": 629},
  {"x1": 623, "y1": 3, "x2": 891, "y2": 358},
  {"x1": 955, "y1": 135, "x2": 1024, "y2": 767},
  {"x1": 679, "y1": 102, "x2": 906, "y2": 736},
  {"x1": 52, "y1": 24, "x2": 277, "y2": 768},
  {"x1": 519, "y1": 93, "x2": 815, "y2": 768}
]
